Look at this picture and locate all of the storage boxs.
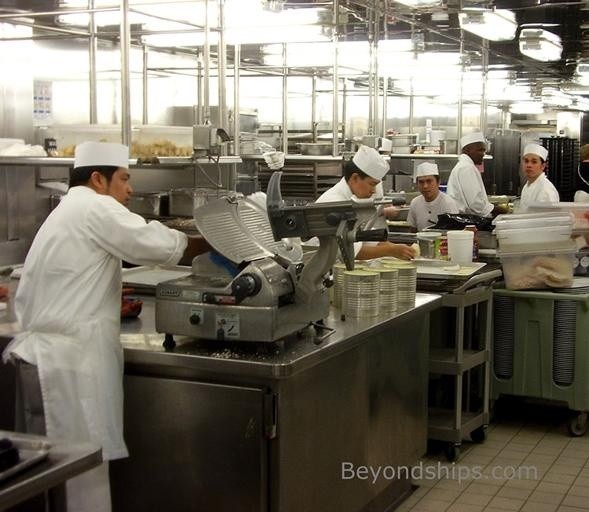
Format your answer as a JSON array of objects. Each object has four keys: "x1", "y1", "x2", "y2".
[
  {"x1": 492, "y1": 201, "x2": 589, "y2": 290},
  {"x1": 58, "y1": 124, "x2": 194, "y2": 161}
]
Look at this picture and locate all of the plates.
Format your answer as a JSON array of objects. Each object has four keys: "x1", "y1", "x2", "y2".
[{"x1": 120, "y1": 265, "x2": 193, "y2": 290}]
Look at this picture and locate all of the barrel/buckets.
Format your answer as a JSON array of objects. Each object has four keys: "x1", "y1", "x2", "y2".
[
  {"x1": 447, "y1": 229, "x2": 475, "y2": 265},
  {"x1": 415, "y1": 231, "x2": 443, "y2": 261}
]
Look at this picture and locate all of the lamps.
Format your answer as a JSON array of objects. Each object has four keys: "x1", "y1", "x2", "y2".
[
  {"x1": 517, "y1": 26, "x2": 564, "y2": 64},
  {"x1": 457, "y1": 5, "x2": 517, "y2": 45}
]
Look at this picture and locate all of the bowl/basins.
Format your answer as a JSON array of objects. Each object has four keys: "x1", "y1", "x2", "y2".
[{"x1": 296, "y1": 142, "x2": 333, "y2": 155}]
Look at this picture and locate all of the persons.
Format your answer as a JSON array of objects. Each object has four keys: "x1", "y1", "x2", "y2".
[
  {"x1": 300, "y1": 145, "x2": 418, "y2": 264},
  {"x1": 447, "y1": 132, "x2": 515, "y2": 217},
  {"x1": 521, "y1": 143, "x2": 559, "y2": 212},
  {"x1": 3, "y1": 143, "x2": 211, "y2": 511},
  {"x1": 573, "y1": 144, "x2": 589, "y2": 203},
  {"x1": 406, "y1": 162, "x2": 459, "y2": 232}
]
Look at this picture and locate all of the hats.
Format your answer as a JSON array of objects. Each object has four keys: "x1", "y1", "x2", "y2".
[
  {"x1": 351, "y1": 145, "x2": 390, "y2": 181},
  {"x1": 414, "y1": 162, "x2": 440, "y2": 176},
  {"x1": 522, "y1": 143, "x2": 549, "y2": 162},
  {"x1": 73, "y1": 141, "x2": 129, "y2": 170},
  {"x1": 459, "y1": 131, "x2": 487, "y2": 150}
]
